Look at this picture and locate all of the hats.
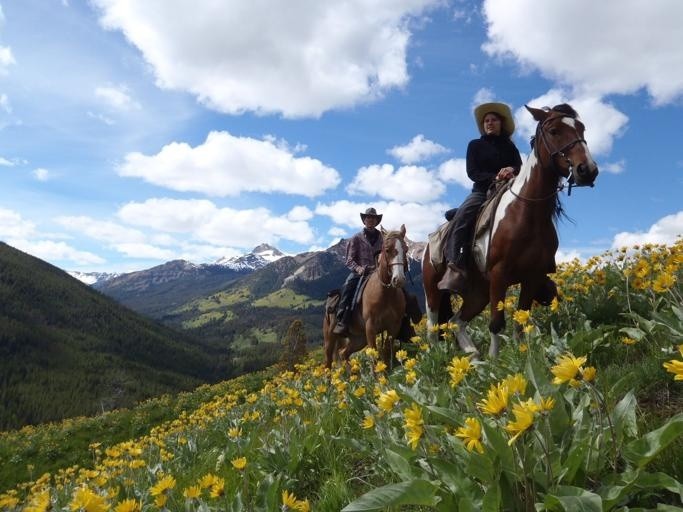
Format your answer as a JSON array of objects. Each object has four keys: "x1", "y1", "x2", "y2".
[
  {"x1": 360, "y1": 208, "x2": 382, "y2": 227},
  {"x1": 474, "y1": 104, "x2": 515, "y2": 139}
]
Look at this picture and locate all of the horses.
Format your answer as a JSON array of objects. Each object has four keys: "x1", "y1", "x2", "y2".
[
  {"x1": 420, "y1": 103, "x2": 599, "y2": 361},
  {"x1": 322, "y1": 223, "x2": 416, "y2": 389}
]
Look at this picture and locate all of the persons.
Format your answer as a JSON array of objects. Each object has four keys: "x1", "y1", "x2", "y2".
[
  {"x1": 434, "y1": 99, "x2": 522, "y2": 296},
  {"x1": 327, "y1": 206, "x2": 385, "y2": 337}
]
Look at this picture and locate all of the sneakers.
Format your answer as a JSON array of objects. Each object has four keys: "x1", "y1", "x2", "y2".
[
  {"x1": 410, "y1": 295, "x2": 421, "y2": 324},
  {"x1": 437, "y1": 263, "x2": 465, "y2": 293}
]
